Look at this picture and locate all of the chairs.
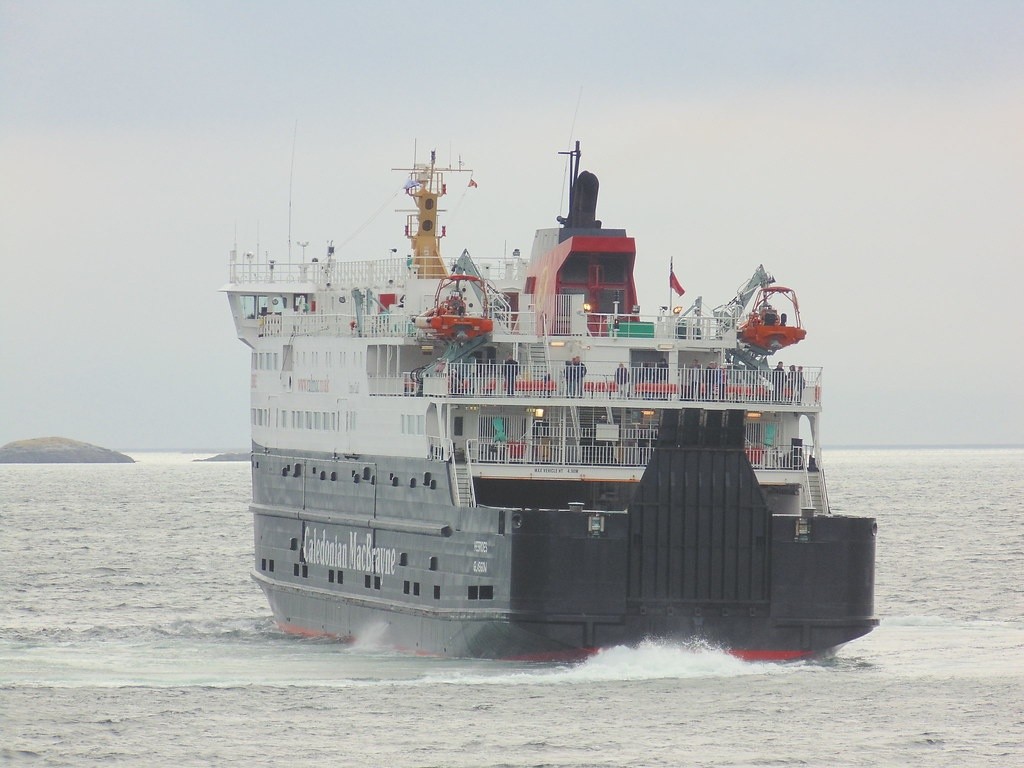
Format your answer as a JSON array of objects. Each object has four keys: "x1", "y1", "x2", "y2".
[{"x1": 402, "y1": 377, "x2": 766, "y2": 401}]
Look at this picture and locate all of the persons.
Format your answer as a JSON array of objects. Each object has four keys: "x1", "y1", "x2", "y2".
[
  {"x1": 689, "y1": 359, "x2": 717, "y2": 402},
  {"x1": 449, "y1": 369, "x2": 463, "y2": 395},
  {"x1": 660, "y1": 359, "x2": 668, "y2": 383},
  {"x1": 596, "y1": 415, "x2": 609, "y2": 425},
  {"x1": 615, "y1": 364, "x2": 630, "y2": 400},
  {"x1": 502, "y1": 354, "x2": 520, "y2": 396},
  {"x1": 564, "y1": 356, "x2": 587, "y2": 398},
  {"x1": 772, "y1": 362, "x2": 805, "y2": 405}
]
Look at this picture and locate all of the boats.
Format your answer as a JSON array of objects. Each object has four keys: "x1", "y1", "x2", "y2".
[{"x1": 218, "y1": 140, "x2": 879, "y2": 665}]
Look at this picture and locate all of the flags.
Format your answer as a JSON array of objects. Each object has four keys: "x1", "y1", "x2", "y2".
[
  {"x1": 467, "y1": 179, "x2": 478, "y2": 188},
  {"x1": 403, "y1": 179, "x2": 419, "y2": 189},
  {"x1": 671, "y1": 272, "x2": 685, "y2": 296}
]
